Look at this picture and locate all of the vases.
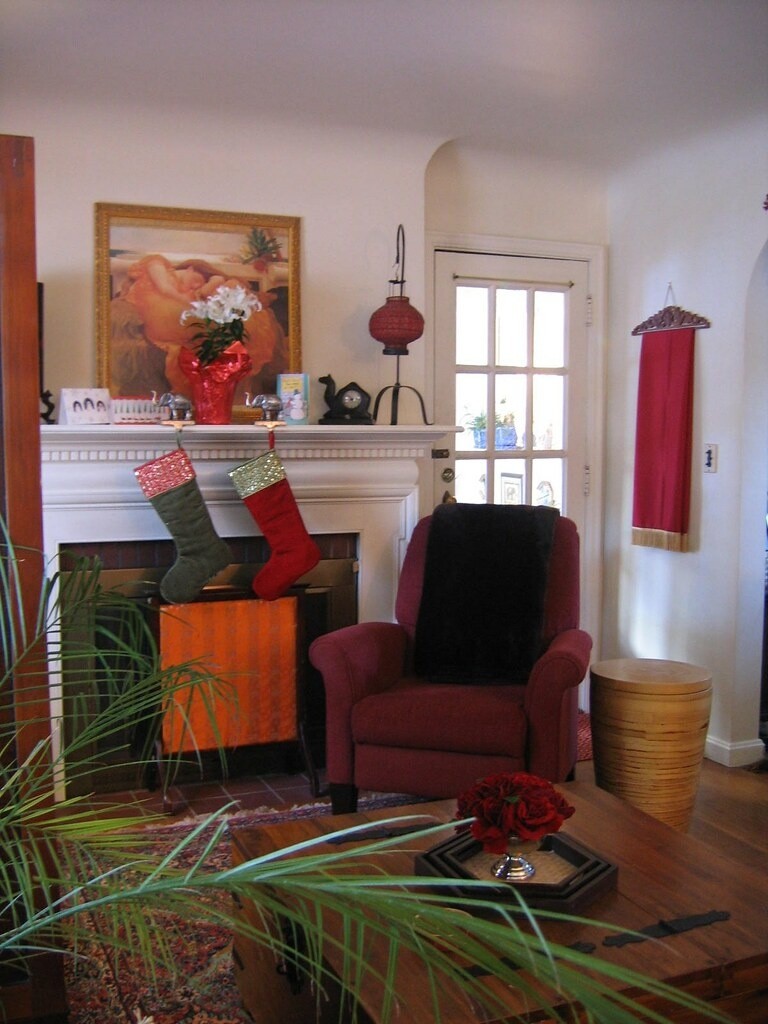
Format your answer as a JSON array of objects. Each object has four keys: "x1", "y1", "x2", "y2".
[
  {"x1": 180, "y1": 346, "x2": 253, "y2": 425},
  {"x1": 505, "y1": 834, "x2": 540, "y2": 855}
]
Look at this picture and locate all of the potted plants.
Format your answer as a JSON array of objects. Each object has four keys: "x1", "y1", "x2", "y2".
[
  {"x1": 1, "y1": 513, "x2": 743, "y2": 1024},
  {"x1": 462, "y1": 407, "x2": 517, "y2": 446}
]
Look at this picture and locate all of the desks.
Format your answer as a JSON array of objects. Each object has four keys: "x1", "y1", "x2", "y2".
[{"x1": 590, "y1": 659, "x2": 713, "y2": 838}]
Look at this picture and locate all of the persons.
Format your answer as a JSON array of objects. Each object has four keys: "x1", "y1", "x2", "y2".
[{"x1": 72, "y1": 397, "x2": 108, "y2": 424}]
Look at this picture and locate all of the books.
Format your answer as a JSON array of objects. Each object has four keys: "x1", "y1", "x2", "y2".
[{"x1": 275, "y1": 373, "x2": 309, "y2": 425}]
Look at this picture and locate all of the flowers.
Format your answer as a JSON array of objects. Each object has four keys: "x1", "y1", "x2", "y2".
[
  {"x1": 180, "y1": 283, "x2": 261, "y2": 367},
  {"x1": 453, "y1": 771, "x2": 577, "y2": 854}
]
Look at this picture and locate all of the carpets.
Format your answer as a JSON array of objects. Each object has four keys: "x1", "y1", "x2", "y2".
[
  {"x1": 53, "y1": 789, "x2": 446, "y2": 1024},
  {"x1": 576, "y1": 713, "x2": 593, "y2": 762}
]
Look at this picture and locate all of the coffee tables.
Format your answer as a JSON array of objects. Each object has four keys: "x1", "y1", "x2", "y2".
[{"x1": 231, "y1": 782, "x2": 768, "y2": 1024}]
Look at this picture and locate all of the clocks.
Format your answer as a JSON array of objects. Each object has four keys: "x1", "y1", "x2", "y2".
[{"x1": 319, "y1": 373, "x2": 374, "y2": 425}]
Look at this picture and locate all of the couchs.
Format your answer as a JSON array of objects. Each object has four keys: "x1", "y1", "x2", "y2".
[{"x1": 309, "y1": 502, "x2": 594, "y2": 816}]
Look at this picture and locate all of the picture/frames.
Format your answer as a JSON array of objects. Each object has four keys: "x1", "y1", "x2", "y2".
[
  {"x1": 501, "y1": 474, "x2": 523, "y2": 503},
  {"x1": 95, "y1": 202, "x2": 302, "y2": 425}
]
